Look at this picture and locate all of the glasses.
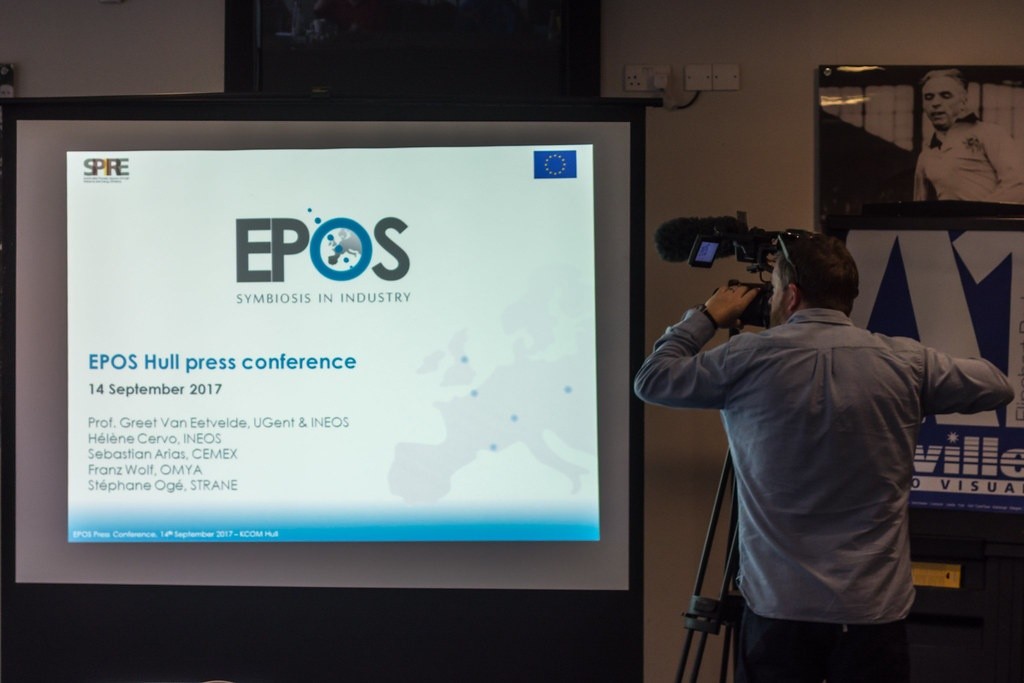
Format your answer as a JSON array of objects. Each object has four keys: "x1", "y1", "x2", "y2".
[{"x1": 776, "y1": 228, "x2": 814, "y2": 286}]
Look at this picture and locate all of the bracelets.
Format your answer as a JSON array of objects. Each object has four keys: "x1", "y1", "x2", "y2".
[{"x1": 698, "y1": 304, "x2": 720, "y2": 330}]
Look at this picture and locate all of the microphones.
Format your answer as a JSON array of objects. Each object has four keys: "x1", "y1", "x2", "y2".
[{"x1": 654, "y1": 216, "x2": 749, "y2": 262}]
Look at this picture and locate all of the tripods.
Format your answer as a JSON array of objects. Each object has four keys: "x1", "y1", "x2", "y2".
[{"x1": 672, "y1": 451, "x2": 738, "y2": 683}]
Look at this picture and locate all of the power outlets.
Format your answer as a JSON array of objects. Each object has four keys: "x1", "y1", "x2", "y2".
[{"x1": 624, "y1": 64, "x2": 647, "y2": 91}]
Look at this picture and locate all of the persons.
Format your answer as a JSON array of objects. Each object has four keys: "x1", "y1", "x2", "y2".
[
  {"x1": 634, "y1": 229, "x2": 1015, "y2": 683},
  {"x1": 906, "y1": 69, "x2": 1024, "y2": 204}
]
